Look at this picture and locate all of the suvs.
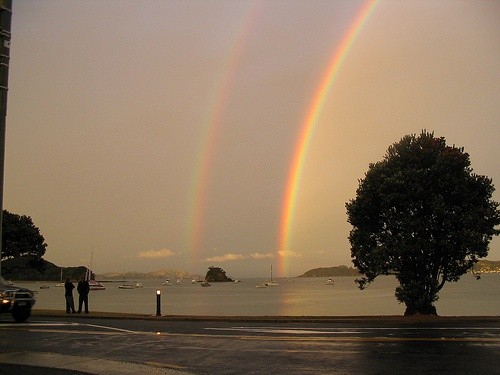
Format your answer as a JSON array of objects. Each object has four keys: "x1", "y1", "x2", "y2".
[{"x1": 0, "y1": 274, "x2": 36, "y2": 322}]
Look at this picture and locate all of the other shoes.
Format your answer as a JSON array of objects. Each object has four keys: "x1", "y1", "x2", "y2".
[
  {"x1": 86, "y1": 311, "x2": 89, "y2": 313},
  {"x1": 67, "y1": 310, "x2": 70, "y2": 313},
  {"x1": 72, "y1": 310, "x2": 76, "y2": 313},
  {"x1": 78, "y1": 311, "x2": 81, "y2": 313}
]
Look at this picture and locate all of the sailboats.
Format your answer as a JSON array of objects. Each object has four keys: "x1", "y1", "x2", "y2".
[
  {"x1": 55, "y1": 269, "x2": 66, "y2": 287},
  {"x1": 84, "y1": 264, "x2": 106, "y2": 290},
  {"x1": 265, "y1": 264, "x2": 279, "y2": 286}
]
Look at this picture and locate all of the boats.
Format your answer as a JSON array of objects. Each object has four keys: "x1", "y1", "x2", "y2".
[
  {"x1": 118, "y1": 284, "x2": 134, "y2": 289},
  {"x1": 326, "y1": 279, "x2": 335, "y2": 285},
  {"x1": 256, "y1": 284, "x2": 267, "y2": 288}
]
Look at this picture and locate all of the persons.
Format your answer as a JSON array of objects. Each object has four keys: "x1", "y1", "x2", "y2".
[
  {"x1": 76, "y1": 276, "x2": 90, "y2": 314},
  {"x1": 64, "y1": 277, "x2": 76, "y2": 314}
]
